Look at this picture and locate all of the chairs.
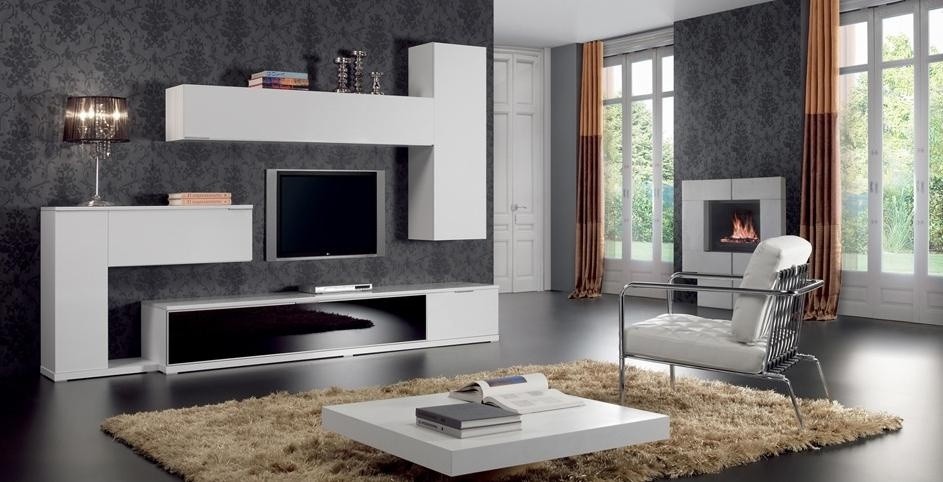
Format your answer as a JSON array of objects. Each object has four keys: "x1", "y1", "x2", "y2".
[{"x1": 613, "y1": 233, "x2": 837, "y2": 430}]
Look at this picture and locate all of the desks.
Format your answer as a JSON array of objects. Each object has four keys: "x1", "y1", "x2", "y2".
[{"x1": 320, "y1": 387, "x2": 674, "y2": 481}]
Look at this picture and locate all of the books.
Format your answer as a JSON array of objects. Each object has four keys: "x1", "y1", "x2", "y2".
[
  {"x1": 415, "y1": 402, "x2": 521, "y2": 439},
  {"x1": 447, "y1": 373, "x2": 585, "y2": 415},
  {"x1": 248, "y1": 70, "x2": 309, "y2": 91},
  {"x1": 167, "y1": 193, "x2": 233, "y2": 205}
]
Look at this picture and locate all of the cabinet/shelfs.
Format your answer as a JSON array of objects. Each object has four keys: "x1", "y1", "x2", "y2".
[
  {"x1": 139, "y1": 283, "x2": 499, "y2": 364},
  {"x1": 107, "y1": 203, "x2": 253, "y2": 268},
  {"x1": 39, "y1": 205, "x2": 109, "y2": 375}
]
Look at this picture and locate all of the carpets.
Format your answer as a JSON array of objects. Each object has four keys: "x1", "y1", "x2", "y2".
[{"x1": 98, "y1": 356, "x2": 906, "y2": 482}]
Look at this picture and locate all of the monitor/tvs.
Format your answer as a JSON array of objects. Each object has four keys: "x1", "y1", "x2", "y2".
[{"x1": 264, "y1": 168, "x2": 386, "y2": 262}]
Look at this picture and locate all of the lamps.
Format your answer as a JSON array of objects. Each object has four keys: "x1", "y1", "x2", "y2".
[{"x1": 64, "y1": 94, "x2": 131, "y2": 208}]
